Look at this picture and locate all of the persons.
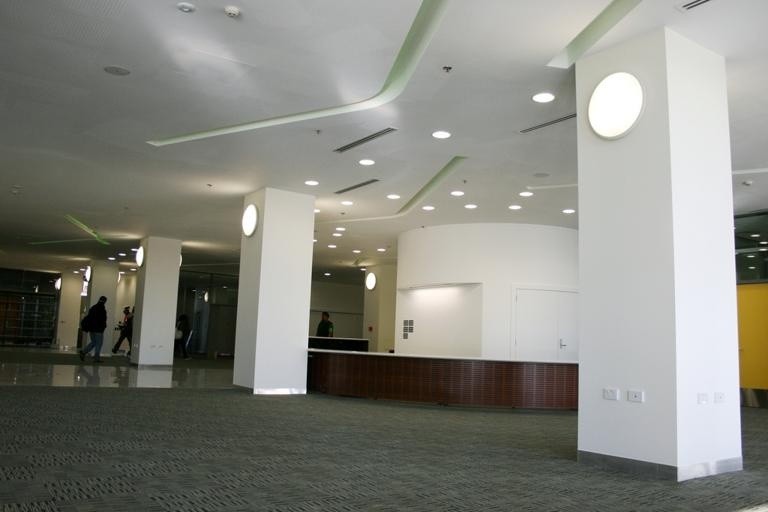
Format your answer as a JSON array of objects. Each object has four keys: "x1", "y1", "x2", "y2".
[
  {"x1": 315, "y1": 311, "x2": 334, "y2": 337},
  {"x1": 80, "y1": 296, "x2": 107, "y2": 362},
  {"x1": 112, "y1": 306, "x2": 134, "y2": 356},
  {"x1": 175, "y1": 315, "x2": 192, "y2": 360}
]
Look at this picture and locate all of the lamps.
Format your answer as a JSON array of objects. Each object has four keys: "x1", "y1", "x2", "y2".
[
  {"x1": 587, "y1": 71, "x2": 645, "y2": 142},
  {"x1": 366, "y1": 273, "x2": 376, "y2": 291},
  {"x1": 136, "y1": 246, "x2": 144, "y2": 267},
  {"x1": 242, "y1": 203, "x2": 258, "y2": 237}
]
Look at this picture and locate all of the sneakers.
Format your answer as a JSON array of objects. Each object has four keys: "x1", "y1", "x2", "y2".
[{"x1": 79, "y1": 350, "x2": 105, "y2": 363}]
